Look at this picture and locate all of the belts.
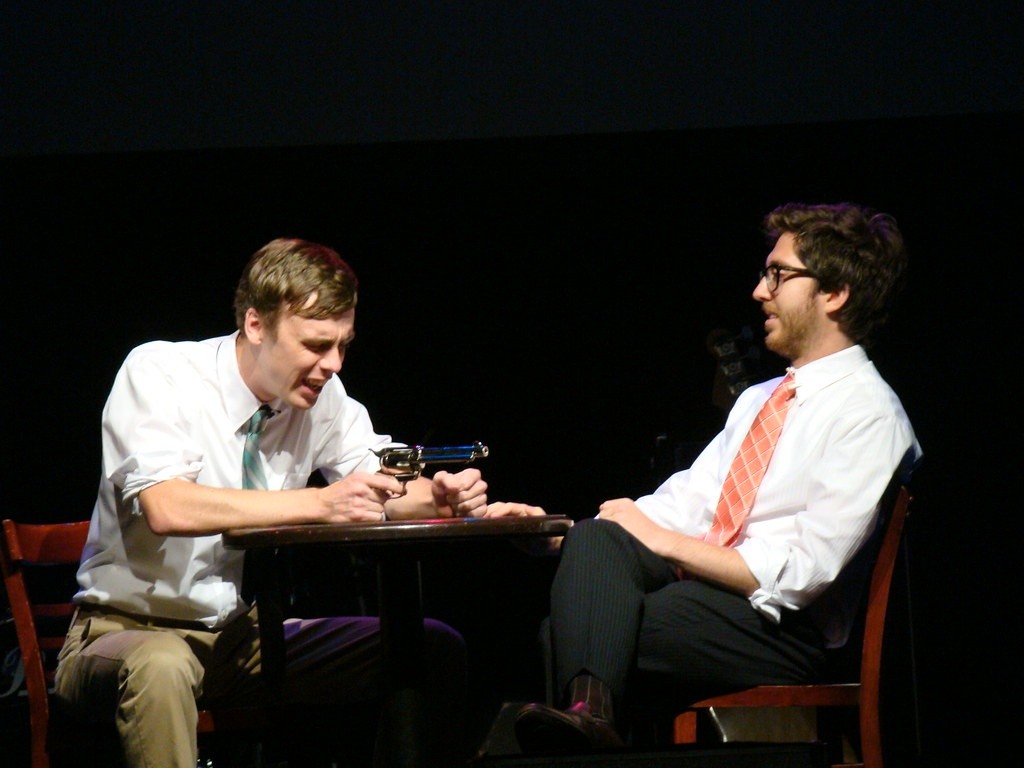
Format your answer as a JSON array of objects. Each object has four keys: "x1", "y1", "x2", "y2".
[{"x1": 81, "y1": 601, "x2": 209, "y2": 633}]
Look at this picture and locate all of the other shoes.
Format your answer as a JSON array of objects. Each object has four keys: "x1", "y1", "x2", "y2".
[{"x1": 515, "y1": 703, "x2": 623, "y2": 754}]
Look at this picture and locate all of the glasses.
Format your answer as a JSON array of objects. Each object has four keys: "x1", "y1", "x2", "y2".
[{"x1": 757, "y1": 264, "x2": 822, "y2": 293}]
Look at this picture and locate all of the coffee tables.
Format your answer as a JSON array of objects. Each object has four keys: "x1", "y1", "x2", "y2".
[{"x1": 223, "y1": 514, "x2": 574, "y2": 768}]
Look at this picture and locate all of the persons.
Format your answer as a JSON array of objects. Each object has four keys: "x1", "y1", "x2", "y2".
[
  {"x1": 484, "y1": 199, "x2": 922, "y2": 767},
  {"x1": 51, "y1": 239, "x2": 489, "y2": 768}
]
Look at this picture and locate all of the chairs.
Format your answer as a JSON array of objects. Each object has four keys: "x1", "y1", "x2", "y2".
[
  {"x1": 0, "y1": 518, "x2": 284, "y2": 768},
  {"x1": 654, "y1": 482, "x2": 911, "y2": 768}
]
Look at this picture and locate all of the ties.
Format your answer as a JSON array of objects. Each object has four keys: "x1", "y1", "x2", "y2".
[
  {"x1": 701, "y1": 375, "x2": 797, "y2": 544},
  {"x1": 242, "y1": 404, "x2": 278, "y2": 489}
]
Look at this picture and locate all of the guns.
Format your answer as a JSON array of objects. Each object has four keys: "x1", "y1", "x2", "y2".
[{"x1": 367, "y1": 439, "x2": 491, "y2": 500}]
